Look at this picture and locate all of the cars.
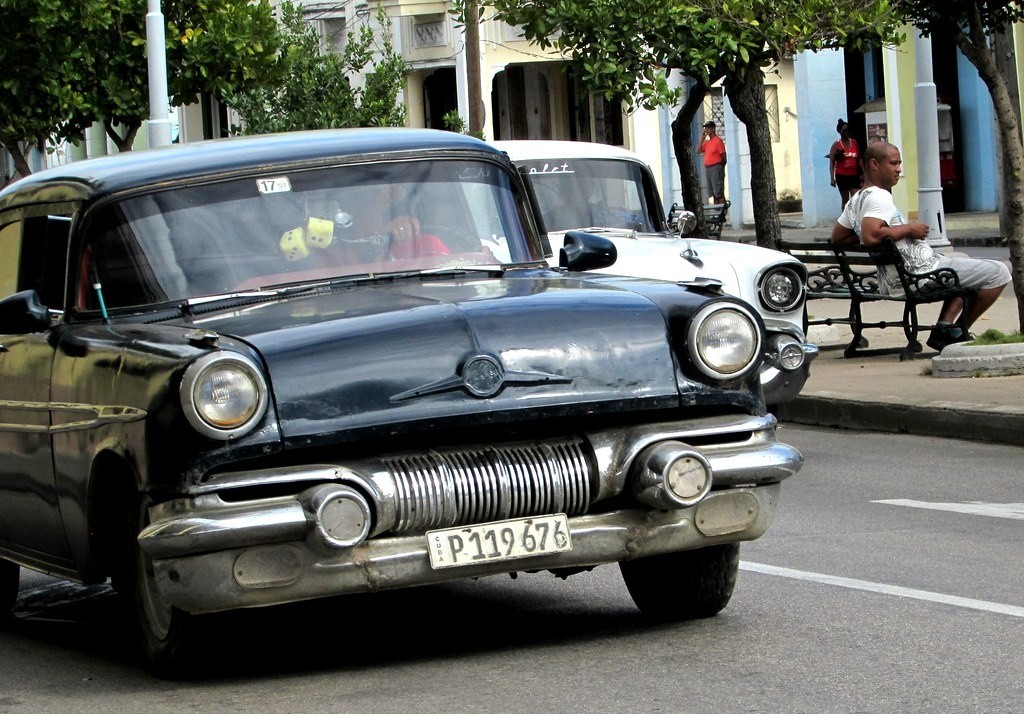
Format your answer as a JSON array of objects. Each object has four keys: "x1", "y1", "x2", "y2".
[
  {"x1": 0, "y1": 119, "x2": 806, "y2": 683},
  {"x1": 483, "y1": 137, "x2": 820, "y2": 413}
]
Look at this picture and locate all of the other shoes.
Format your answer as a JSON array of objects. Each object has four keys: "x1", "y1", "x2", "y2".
[
  {"x1": 926, "y1": 323, "x2": 974, "y2": 352},
  {"x1": 710, "y1": 216, "x2": 726, "y2": 222}
]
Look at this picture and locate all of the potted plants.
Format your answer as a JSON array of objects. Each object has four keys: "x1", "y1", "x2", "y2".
[{"x1": 780, "y1": 189, "x2": 802, "y2": 212}]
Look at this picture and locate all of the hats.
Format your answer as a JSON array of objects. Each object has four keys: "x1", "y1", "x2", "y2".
[{"x1": 702, "y1": 121, "x2": 715, "y2": 127}]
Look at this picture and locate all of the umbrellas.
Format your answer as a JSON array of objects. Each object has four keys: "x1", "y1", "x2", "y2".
[{"x1": 823, "y1": 149, "x2": 848, "y2": 162}]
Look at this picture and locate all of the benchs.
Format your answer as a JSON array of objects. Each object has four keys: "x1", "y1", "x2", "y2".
[
  {"x1": 775, "y1": 237, "x2": 979, "y2": 361},
  {"x1": 666, "y1": 200, "x2": 731, "y2": 241}
]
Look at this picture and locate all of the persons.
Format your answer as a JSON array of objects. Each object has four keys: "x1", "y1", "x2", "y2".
[
  {"x1": 695, "y1": 120, "x2": 727, "y2": 223},
  {"x1": 829, "y1": 142, "x2": 1012, "y2": 352},
  {"x1": 824, "y1": 119, "x2": 866, "y2": 213},
  {"x1": 542, "y1": 175, "x2": 617, "y2": 233},
  {"x1": 335, "y1": 171, "x2": 452, "y2": 260}
]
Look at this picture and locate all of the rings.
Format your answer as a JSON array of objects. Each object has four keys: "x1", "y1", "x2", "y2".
[{"x1": 400, "y1": 228, "x2": 404, "y2": 230}]
